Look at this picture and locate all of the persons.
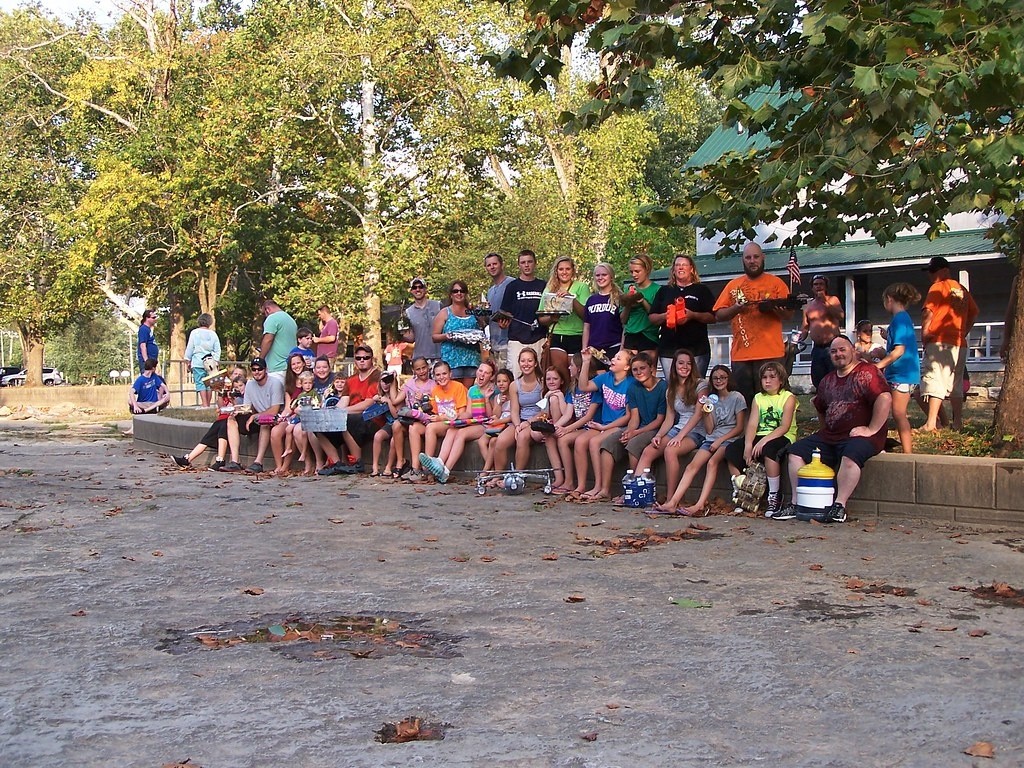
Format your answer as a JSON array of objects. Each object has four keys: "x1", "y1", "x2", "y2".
[
  {"x1": 128, "y1": 358, "x2": 170, "y2": 414},
  {"x1": 121, "y1": 368, "x2": 169, "y2": 437},
  {"x1": 137, "y1": 310, "x2": 159, "y2": 375},
  {"x1": 169, "y1": 239, "x2": 979, "y2": 522}
]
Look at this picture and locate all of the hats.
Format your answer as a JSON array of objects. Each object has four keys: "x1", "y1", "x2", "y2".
[
  {"x1": 921, "y1": 257, "x2": 950, "y2": 273},
  {"x1": 410, "y1": 277, "x2": 427, "y2": 287},
  {"x1": 250, "y1": 357, "x2": 266, "y2": 368},
  {"x1": 355, "y1": 344, "x2": 372, "y2": 355}
]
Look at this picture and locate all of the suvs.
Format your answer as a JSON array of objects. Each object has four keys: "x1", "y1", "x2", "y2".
[
  {"x1": 2, "y1": 367, "x2": 62, "y2": 387},
  {"x1": 0, "y1": 367, "x2": 21, "y2": 387}
]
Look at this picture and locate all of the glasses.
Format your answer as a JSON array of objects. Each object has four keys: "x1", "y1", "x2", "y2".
[
  {"x1": 412, "y1": 286, "x2": 425, "y2": 289},
  {"x1": 355, "y1": 355, "x2": 372, "y2": 360},
  {"x1": 711, "y1": 375, "x2": 728, "y2": 381},
  {"x1": 252, "y1": 367, "x2": 265, "y2": 371},
  {"x1": 450, "y1": 289, "x2": 467, "y2": 293}
]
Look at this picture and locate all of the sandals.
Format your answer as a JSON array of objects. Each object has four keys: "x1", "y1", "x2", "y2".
[{"x1": 317, "y1": 452, "x2": 710, "y2": 517}]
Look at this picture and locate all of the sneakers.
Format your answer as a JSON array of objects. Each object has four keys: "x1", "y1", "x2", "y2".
[
  {"x1": 824, "y1": 501, "x2": 847, "y2": 522},
  {"x1": 245, "y1": 462, "x2": 263, "y2": 473},
  {"x1": 733, "y1": 492, "x2": 744, "y2": 513},
  {"x1": 771, "y1": 502, "x2": 797, "y2": 519},
  {"x1": 765, "y1": 489, "x2": 785, "y2": 518},
  {"x1": 169, "y1": 453, "x2": 190, "y2": 468},
  {"x1": 207, "y1": 456, "x2": 225, "y2": 471},
  {"x1": 219, "y1": 461, "x2": 243, "y2": 472}
]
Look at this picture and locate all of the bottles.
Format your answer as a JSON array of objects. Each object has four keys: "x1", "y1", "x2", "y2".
[
  {"x1": 638, "y1": 468, "x2": 656, "y2": 507},
  {"x1": 588, "y1": 345, "x2": 612, "y2": 366},
  {"x1": 730, "y1": 462, "x2": 766, "y2": 512},
  {"x1": 620, "y1": 469, "x2": 639, "y2": 507},
  {"x1": 797, "y1": 449, "x2": 836, "y2": 522},
  {"x1": 214, "y1": 404, "x2": 251, "y2": 413}
]
